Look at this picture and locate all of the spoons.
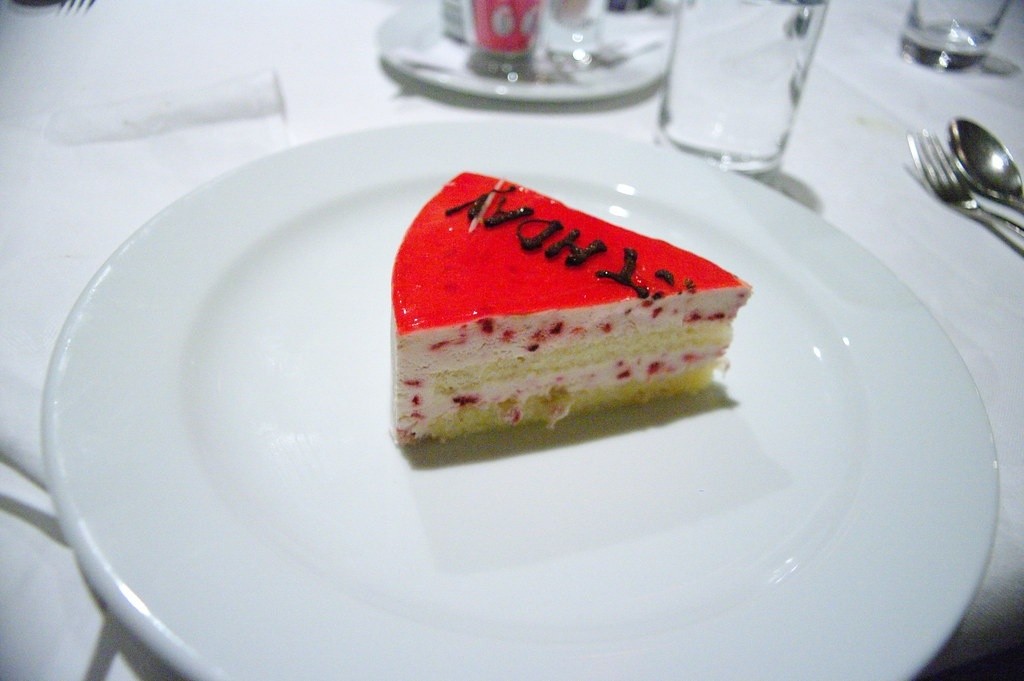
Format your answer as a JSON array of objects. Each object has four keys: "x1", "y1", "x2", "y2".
[{"x1": 946, "y1": 118, "x2": 1024, "y2": 205}]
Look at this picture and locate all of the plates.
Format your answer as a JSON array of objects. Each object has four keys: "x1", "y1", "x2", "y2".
[
  {"x1": 43, "y1": 121, "x2": 1000, "y2": 681},
  {"x1": 378, "y1": 0, "x2": 668, "y2": 102}
]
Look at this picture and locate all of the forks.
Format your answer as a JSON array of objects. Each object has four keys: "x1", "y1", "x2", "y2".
[{"x1": 907, "y1": 133, "x2": 1024, "y2": 235}]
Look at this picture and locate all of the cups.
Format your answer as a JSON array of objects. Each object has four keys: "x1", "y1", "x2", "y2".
[
  {"x1": 656, "y1": 0, "x2": 827, "y2": 183},
  {"x1": 902, "y1": 0, "x2": 1010, "y2": 70},
  {"x1": 466, "y1": 0, "x2": 538, "y2": 81}
]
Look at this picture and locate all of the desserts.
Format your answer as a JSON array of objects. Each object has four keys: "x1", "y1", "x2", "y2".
[{"x1": 391, "y1": 172, "x2": 754, "y2": 443}]
